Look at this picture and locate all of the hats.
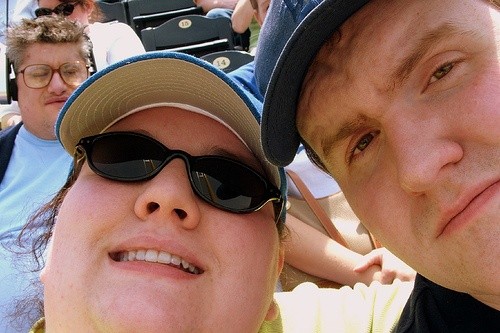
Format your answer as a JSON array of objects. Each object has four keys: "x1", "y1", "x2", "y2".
[
  {"x1": 53, "y1": 52, "x2": 288, "y2": 194},
  {"x1": 254, "y1": 0, "x2": 324, "y2": 167}
]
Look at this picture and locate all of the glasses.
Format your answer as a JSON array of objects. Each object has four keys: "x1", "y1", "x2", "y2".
[
  {"x1": 34, "y1": 3, "x2": 79, "y2": 19},
  {"x1": 16, "y1": 63, "x2": 91, "y2": 89},
  {"x1": 71, "y1": 132, "x2": 285, "y2": 213}
]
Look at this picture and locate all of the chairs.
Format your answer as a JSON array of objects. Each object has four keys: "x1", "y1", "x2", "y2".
[{"x1": 96, "y1": 0, "x2": 255, "y2": 74}]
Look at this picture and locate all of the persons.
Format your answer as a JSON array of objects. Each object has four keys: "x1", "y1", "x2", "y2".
[
  {"x1": 2, "y1": 50, "x2": 414, "y2": 333},
  {"x1": 0, "y1": 16, "x2": 98, "y2": 333},
  {"x1": 253, "y1": 0, "x2": 500, "y2": 333},
  {"x1": 0, "y1": 0, "x2": 414, "y2": 291}
]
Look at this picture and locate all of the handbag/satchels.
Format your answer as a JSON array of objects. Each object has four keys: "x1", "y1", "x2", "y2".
[{"x1": 278, "y1": 170, "x2": 386, "y2": 292}]
